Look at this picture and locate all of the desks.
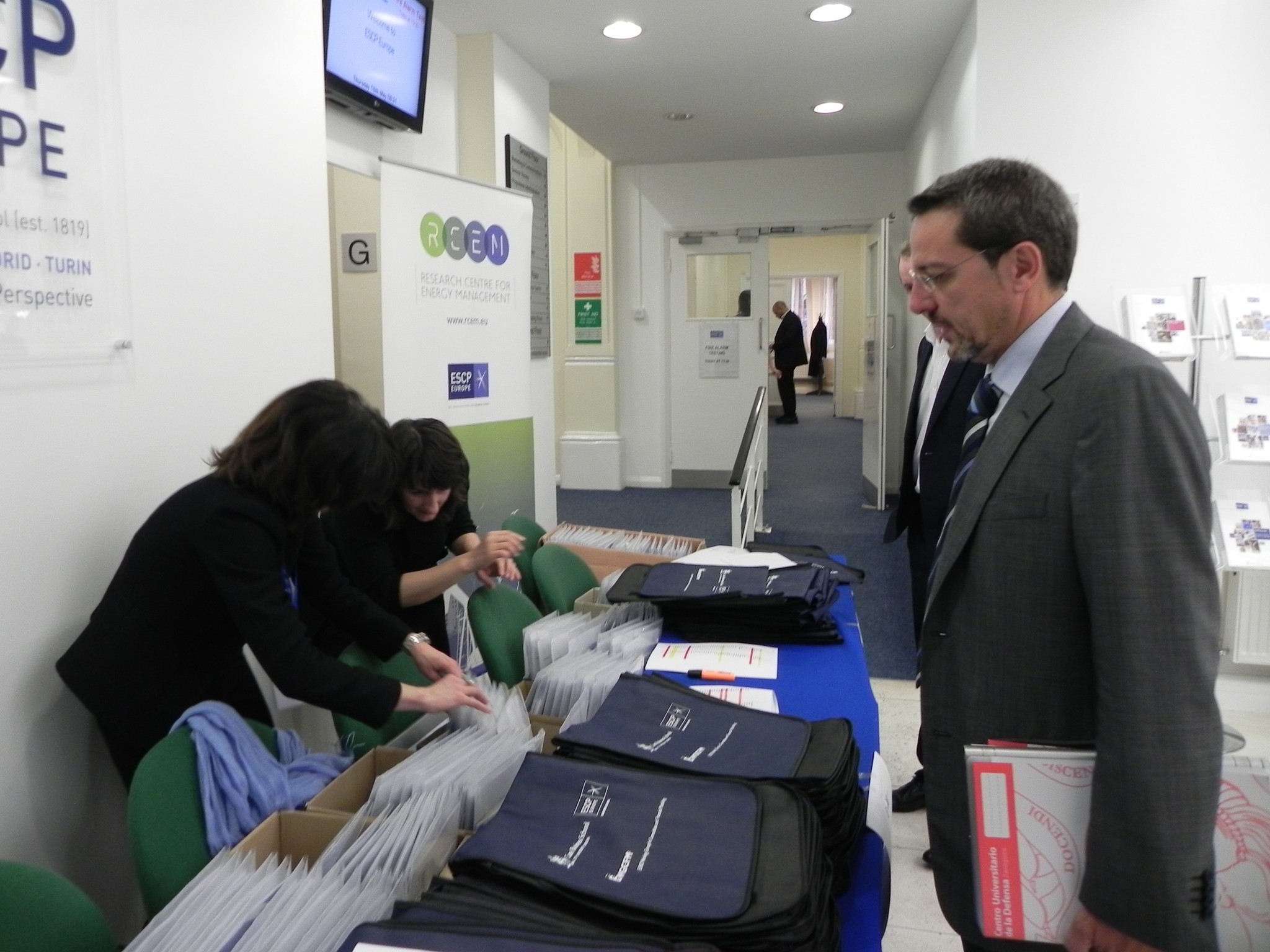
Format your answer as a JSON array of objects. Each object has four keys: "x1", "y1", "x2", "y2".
[{"x1": 436, "y1": 551, "x2": 882, "y2": 952}]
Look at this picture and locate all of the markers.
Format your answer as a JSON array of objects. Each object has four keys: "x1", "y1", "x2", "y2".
[{"x1": 687, "y1": 670, "x2": 736, "y2": 682}]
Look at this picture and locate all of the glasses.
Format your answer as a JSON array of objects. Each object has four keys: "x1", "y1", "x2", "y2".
[{"x1": 908, "y1": 248, "x2": 990, "y2": 293}]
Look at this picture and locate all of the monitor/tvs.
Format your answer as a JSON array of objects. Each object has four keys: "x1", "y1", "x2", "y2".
[{"x1": 323, "y1": 0, "x2": 436, "y2": 133}]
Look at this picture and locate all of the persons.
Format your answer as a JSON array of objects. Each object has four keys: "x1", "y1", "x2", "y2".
[
  {"x1": 771, "y1": 300, "x2": 808, "y2": 427},
  {"x1": 883, "y1": 239, "x2": 985, "y2": 864},
  {"x1": 737, "y1": 290, "x2": 751, "y2": 317},
  {"x1": 388, "y1": 419, "x2": 527, "y2": 668},
  {"x1": 905, "y1": 158, "x2": 1221, "y2": 951},
  {"x1": 55, "y1": 376, "x2": 493, "y2": 798}
]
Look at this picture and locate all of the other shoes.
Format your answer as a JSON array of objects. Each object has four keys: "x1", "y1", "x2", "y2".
[
  {"x1": 774, "y1": 414, "x2": 798, "y2": 425},
  {"x1": 891, "y1": 768, "x2": 927, "y2": 812},
  {"x1": 922, "y1": 849, "x2": 932, "y2": 864}
]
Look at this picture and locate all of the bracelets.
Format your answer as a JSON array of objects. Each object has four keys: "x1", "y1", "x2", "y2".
[{"x1": 403, "y1": 631, "x2": 430, "y2": 653}]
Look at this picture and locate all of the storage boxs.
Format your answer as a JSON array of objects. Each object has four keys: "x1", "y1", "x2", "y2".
[
  {"x1": 541, "y1": 521, "x2": 706, "y2": 567},
  {"x1": 572, "y1": 586, "x2": 643, "y2": 620},
  {"x1": 306, "y1": 743, "x2": 477, "y2": 836},
  {"x1": 230, "y1": 810, "x2": 377, "y2": 869},
  {"x1": 509, "y1": 679, "x2": 566, "y2": 727}
]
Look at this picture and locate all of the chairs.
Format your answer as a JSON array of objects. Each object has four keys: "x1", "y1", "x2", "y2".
[
  {"x1": 0, "y1": 859, "x2": 120, "y2": 952},
  {"x1": 124, "y1": 698, "x2": 356, "y2": 916},
  {"x1": 332, "y1": 642, "x2": 435, "y2": 758},
  {"x1": 531, "y1": 543, "x2": 600, "y2": 615},
  {"x1": 467, "y1": 583, "x2": 547, "y2": 688},
  {"x1": 501, "y1": 514, "x2": 546, "y2": 613}
]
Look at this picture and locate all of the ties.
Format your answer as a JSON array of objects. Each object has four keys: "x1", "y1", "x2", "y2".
[{"x1": 915, "y1": 374, "x2": 1004, "y2": 690}]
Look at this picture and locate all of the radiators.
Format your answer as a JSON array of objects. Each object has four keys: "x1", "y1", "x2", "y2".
[{"x1": 1230, "y1": 569, "x2": 1270, "y2": 667}]
